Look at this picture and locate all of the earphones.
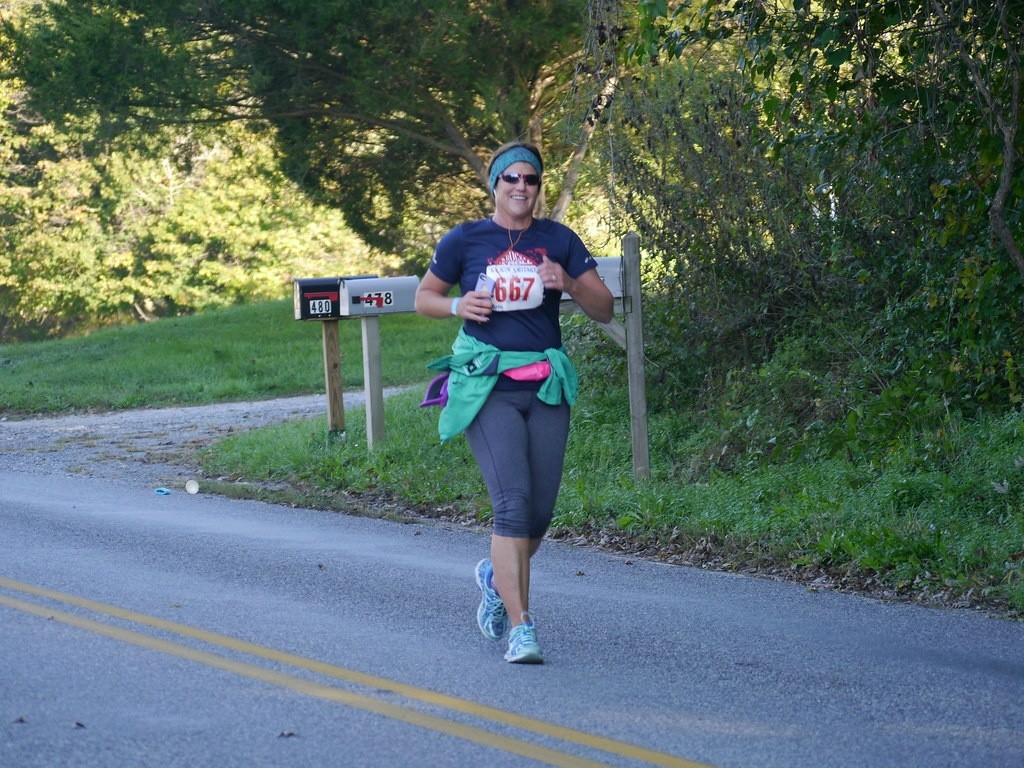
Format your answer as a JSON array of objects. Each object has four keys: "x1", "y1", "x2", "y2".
[{"x1": 493, "y1": 189, "x2": 496, "y2": 195}]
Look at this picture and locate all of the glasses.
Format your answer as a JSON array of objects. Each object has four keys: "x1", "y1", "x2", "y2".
[{"x1": 499, "y1": 172, "x2": 540, "y2": 185}]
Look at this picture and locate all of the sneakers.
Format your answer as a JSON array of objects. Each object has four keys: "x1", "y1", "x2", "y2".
[
  {"x1": 474, "y1": 558, "x2": 507, "y2": 640},
  {"x1": 504, "y1": 612, "x2": 544, "y2": 661}
]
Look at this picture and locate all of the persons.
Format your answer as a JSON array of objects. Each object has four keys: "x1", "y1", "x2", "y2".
[{"x1": 414, "y1": 142, "x2": 614, "y2": 664}]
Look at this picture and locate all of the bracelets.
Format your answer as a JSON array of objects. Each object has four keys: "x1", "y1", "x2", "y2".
[{"x1": 451, "y1": 297, "x2": 460, "y2": 316}]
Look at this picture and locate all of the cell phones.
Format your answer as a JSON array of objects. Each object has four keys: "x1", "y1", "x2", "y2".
[{"x1": 475, "y1": 271, "x2": 495, "y2": 325}]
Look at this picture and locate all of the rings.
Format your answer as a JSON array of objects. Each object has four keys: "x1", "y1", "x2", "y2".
[{"x1": 552, "y1": 274, "x2": 557, "y2": 282}]
